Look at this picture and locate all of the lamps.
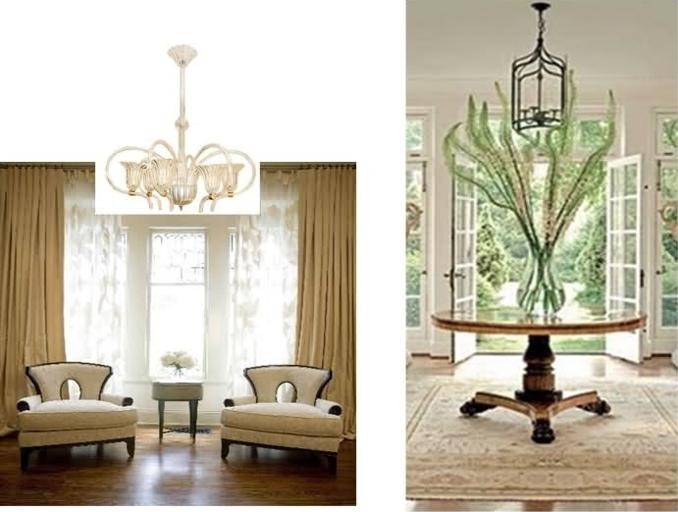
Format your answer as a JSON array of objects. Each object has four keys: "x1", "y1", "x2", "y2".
[
  {"x1": 508, "y1": 1, "x2": 570, "y2": 141},
  {"x1": 105, "y1": 46, "x2": 255, "y2": 212}
]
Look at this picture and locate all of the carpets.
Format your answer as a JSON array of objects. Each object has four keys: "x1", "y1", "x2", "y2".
[{"x1": 405, "y1": 375, "x2": 678, "y2": 501}]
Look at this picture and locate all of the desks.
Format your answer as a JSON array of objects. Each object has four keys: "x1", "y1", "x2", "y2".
[
  {"x1": 152, "y1": 378, "x2": 204, "y2": 444},
  {"x1": 430, "y1": 307, "x2": 648, "y2": 442}
]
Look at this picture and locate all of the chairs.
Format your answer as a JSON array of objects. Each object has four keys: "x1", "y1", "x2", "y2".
[
  {"x1": 12, "y1": 361, "x2": 138, "y2": 472},
  {"x1": 219, "y1": 366, "x2": 346, "y2": 475}
]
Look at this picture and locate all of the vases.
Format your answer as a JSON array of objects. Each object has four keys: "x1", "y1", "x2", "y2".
[{"x1": 517, "y1": 243, "x2": 567, "y2": 317}]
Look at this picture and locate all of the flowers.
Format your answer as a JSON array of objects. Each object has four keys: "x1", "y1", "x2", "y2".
[
  {"x1": 158, "y1": 345, "x2": 197, "y2": 377},
  {"x1": 439, "y1": 80, "x2": 618, "y2": 313}
]
